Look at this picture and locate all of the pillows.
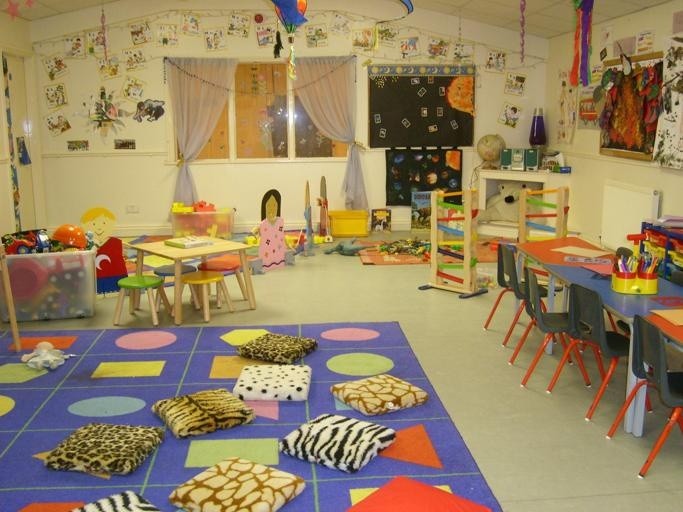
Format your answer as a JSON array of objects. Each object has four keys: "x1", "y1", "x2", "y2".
[
  {"x1": 233, "y1": 365, "x2": 311, "y2": 401},
  {"x1": 278, "y1": 413, "x2": 396, "y2": 473},
  {"x1": 330, "y1": 373, "x2": 429, "y2": 416},
  {"x1": 169, "y1": 456, "x2": 305, "y2": 512},
  {"x1": 236, "y1": 333, "x2": 317, "y2": 365},
  {"x1": 151, "y1": 388, "x2": 256, "y2": 439},
  {"x1": 44, "y1": 422, "x2": 162, "y2": 475},
  {"x1": 71, "y1": 491, "x2": 161, "y2": 512}
]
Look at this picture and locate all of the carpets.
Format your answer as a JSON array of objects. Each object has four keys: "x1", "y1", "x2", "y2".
[
  {"x1": 1, "y1": 321, "x2": 504, "y2": 512},
  {"x1": 353, "y1": 237, "x2": 515, "y2": 265}
]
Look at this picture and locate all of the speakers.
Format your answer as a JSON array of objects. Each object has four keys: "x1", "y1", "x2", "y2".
[
  {"x1": 525, "y1": 148, "x2": 539, "y2": 171},
  {"x1": 501, "y1": 149, "x2": 512, "y2": 170}
]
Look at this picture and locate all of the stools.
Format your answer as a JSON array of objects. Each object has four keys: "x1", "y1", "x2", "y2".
[
  {"x1": 170, "y1": 271, "x2": 235, "y2": 323},
  {"x1": 113, "y1": 276, "x2": 171, "y2": 327},
  {"x1": 197, "y1": 260, "x2": 248, "y2": 308},
  {"x1": 154, "y1": 264, "x2": 196, "y2": 313}
]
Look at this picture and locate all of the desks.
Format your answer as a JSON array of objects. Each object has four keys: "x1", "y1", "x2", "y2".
[
  {"x1": 478, "y1": 168, "x2": 569, "y2": 227},
  {"x1": 130, "y1": 237, "x2": 258, "y2": 325}
]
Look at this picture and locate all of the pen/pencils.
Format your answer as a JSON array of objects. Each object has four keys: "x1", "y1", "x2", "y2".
[{"x1": 613, "y1": 254, "x2": 663, "y2": 273}]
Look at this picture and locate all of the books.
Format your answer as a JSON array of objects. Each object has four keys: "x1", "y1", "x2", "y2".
[{"x1": 164, "y1": 234, "x2": 212, "y2": 249}]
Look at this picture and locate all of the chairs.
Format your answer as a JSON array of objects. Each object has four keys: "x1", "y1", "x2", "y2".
[
  {"x1": 518, "y1": 185, "x2": 569, "y2": 283},
  {"x1": 419, "y1": 189, "x2": 488, "y2": 298}
]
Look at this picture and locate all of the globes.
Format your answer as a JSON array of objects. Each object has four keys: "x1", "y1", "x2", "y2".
[{"x1": 477, "y1": 134, "x2": 505, "y2": 170}]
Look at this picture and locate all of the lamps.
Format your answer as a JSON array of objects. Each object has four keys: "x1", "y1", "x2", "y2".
[{"x1": 528, "y1": 108, "x2": 547, "y2": 151}]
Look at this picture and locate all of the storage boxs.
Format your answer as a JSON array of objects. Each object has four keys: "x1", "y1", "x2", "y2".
[
  {"x1": 328, "y1": 209, "x2": 370, "y2": 237},
  {"x1": 1, "y1": 246, "x2": 97, "y2": 323},
  {"x1": 170, "y1": 206, "x2": 237, "y2": 241}
]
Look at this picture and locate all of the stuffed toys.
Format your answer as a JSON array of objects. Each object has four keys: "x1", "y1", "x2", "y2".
[{"x1": 472, "y1": 179, "x2": 547, "y2": 226}]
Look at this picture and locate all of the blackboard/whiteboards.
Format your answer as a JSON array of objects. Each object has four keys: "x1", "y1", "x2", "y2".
[{"x1": 366, "y1": 63, "x2": 475, "y2": 148}]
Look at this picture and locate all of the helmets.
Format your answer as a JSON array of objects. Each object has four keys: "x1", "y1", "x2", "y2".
[{"x1": 52, "y1": 225, "x2": 86, "y2": 247}]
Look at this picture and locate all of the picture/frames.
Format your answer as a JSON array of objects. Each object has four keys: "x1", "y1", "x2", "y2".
[{"x1": 599, "y1": 51, "x2": 663, "y2": 162}]
